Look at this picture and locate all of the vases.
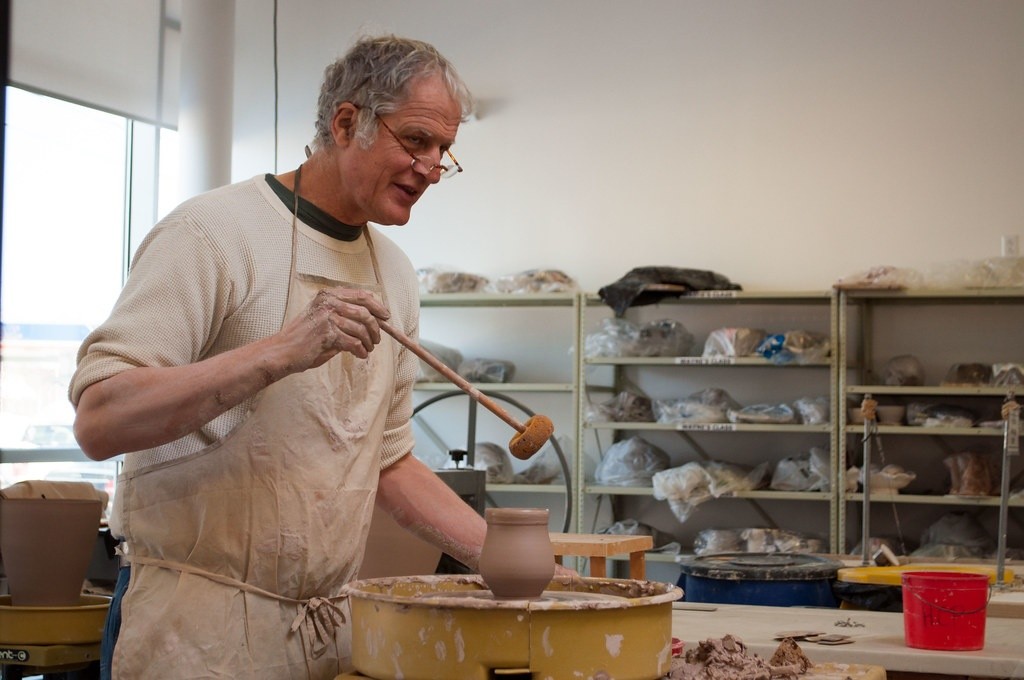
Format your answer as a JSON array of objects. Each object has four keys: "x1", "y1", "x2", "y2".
[{"x1": 0, "y1": 498, "x2": 103, "y2": 607}]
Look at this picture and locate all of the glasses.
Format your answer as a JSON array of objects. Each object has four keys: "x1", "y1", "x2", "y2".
[{"x1": 354, "y1": 106, "x2": 463, "y2": 179}]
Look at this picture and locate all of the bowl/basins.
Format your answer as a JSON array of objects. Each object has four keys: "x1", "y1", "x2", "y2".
[
  {"x1": 875, "y1": 405, "x2": 905, "y2": 425},
  {"x1": 857, "y1": 470, "x2": 916, "y2": 494},
  {"x1": 847, "y1": 408, "x2": 865, "y2": 425}
]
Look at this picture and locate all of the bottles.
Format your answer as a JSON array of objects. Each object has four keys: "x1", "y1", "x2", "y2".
[{"x1": 478, "y1": 507, "x2": 556, "y2": 600}]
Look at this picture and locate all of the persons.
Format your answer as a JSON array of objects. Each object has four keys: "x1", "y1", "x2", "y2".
[{"x1": 67, "y1": 32, "x2": 577, "y2": 680}]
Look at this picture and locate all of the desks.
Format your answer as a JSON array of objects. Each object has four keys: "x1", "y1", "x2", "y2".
[{"x1": 672, "y1": 601, "x2": 1024, "y2": 680}]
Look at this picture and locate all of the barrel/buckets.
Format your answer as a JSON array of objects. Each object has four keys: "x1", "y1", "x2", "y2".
[
  {"x1": 901, "y1": 572, "x2": 992, "y2": 651},
  {"x1": 680, "y1": 551, "x2": 845, "y2": 607}
]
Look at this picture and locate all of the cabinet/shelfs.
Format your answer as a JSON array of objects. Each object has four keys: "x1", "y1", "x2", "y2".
[{"x1": 413, "y1": 288, "x2": 1023, "y2": 580}]
